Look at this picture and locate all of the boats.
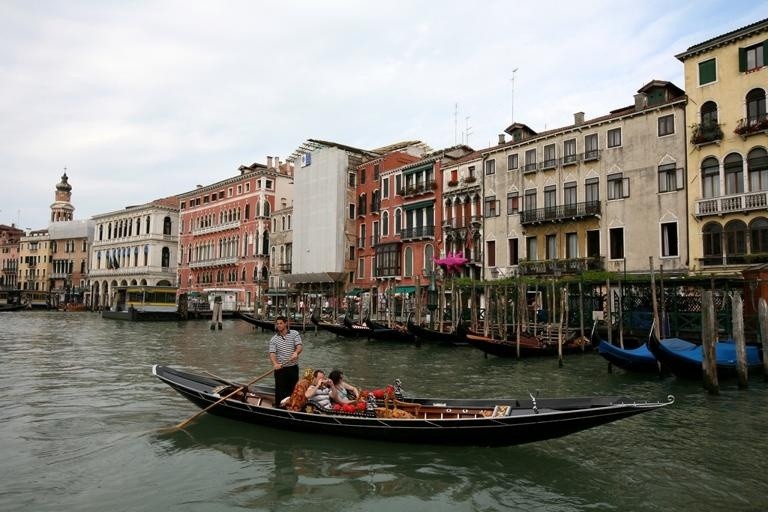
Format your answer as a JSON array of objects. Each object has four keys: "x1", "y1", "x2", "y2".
[{"x1": 146, "y1": 364, "x2": 676, "y2": 448}]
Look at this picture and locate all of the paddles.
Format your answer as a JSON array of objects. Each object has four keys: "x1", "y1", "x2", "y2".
[
  {"x1": 192, "y1": 369, "x2": 240, "y2": 388},
  {"x1": 177, "y1": 359, "x2": 291, "y2": 428}
]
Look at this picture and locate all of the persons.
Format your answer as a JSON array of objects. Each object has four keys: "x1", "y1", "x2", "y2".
[
  {"x1": 326, "y1": 370, "x2": 368, "y2": 412},
  {"x1": 267, "y1": 297, "x2": 272, "y2": 313},
  {"x1": 268, "y1": 316, "x2": 304, "y2": 410},
  {"x1": 266, "y1": 431, "x2": 302, "y2": 505},
  {"x1": 323, "y1": 300, "x2": 329, "y2": 307},
  {"x1": 304, "y1": 369, "x2": 339, "y2": 411},
  {"x1": 526, "y1": 297, "x2": 546, "y2": 322},
  {"x1": 298, "y1": 300, "x2": 304, "y2": 313}
]
